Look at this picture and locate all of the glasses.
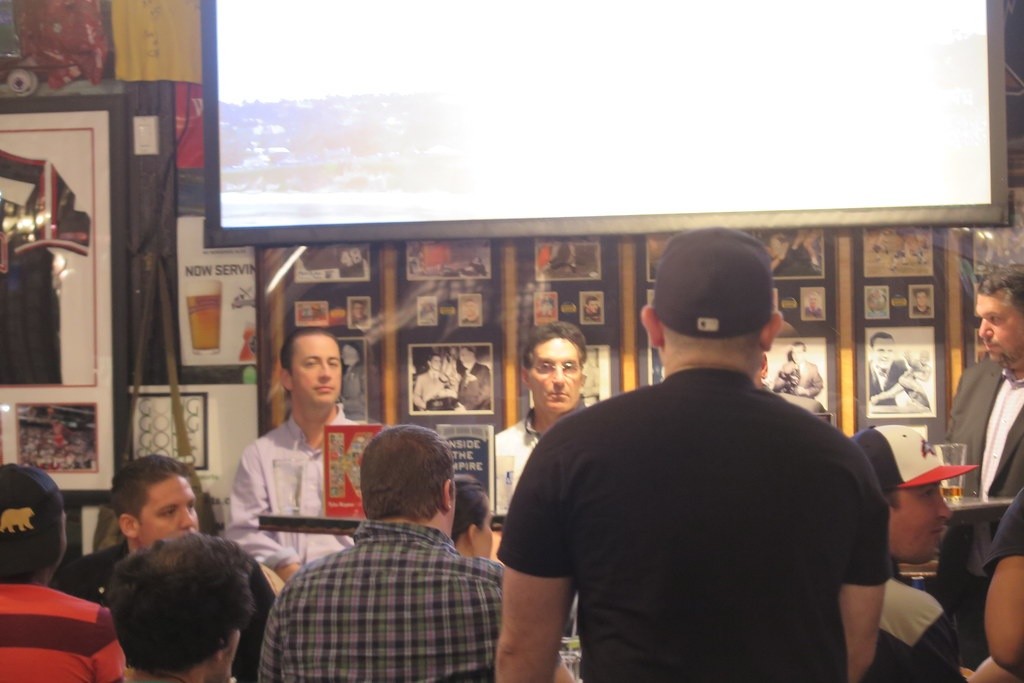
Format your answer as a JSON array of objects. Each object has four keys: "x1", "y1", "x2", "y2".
[{"x1": 532, "y1": 362, "x2": 580, "y2": 376}]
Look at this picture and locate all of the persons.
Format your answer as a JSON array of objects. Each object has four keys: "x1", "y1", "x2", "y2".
[
  {"x1": 868, "y1": 331, "x2": 932, "y2": 414},
  {"x1": 851, "y1": 425, "x2": 1024, "y2": 683},
  {"x1": 540, "y1": 294, "x2": 555, "y2": 317},
  {"x1": 258, "y1": 425, "x2": 572, "y2": 683},
  {"x1": 805, "y1": 292, "x2": 823, "y2": 320},
  {"x1": 340, "y1": 342, "x2": 366, "y2": 421},
  {"x1": 913, "y1": 288, "x2": 930, "y2": 316},
  {"x1": 496, "y1": 228, "x2": 890, "y2": 683},
  {"x1": 765, "y1": 227, "x2": 821, "y2": 276},
  {"x1": 352, "y1": 299, "x2": 371, "y2": 327},
  {"x1": 583, "y1": 297, "x2": 603, "y2": 323},
  {"x1": 868, "y1": 287, "x2": 886, "y2": 317},
  {"x1": 772, "y1": 340, "x2": 824, "y2": 398},
  {"x1": 869, "y1": 227, "x2": 928, "y2": 273},
  {"x1": 941, "y1": 265, "x2": 1024, "y2": 498},
  {"x1": 227, "y1": 329, "x2": 382, "y2": 585},
  {"x1": 0, "y1": 463, "x2": 125, "y2": 683},
  {"x1": 59, "y1": 455, "x2": 275, "y2": 683},
  {"x1": 459, "y1": 298, "x2": 482, "y2": 326},
  {"x1": 540, "y1": 241, "x2": 577, "y2": 277},
  {"x1": 19, "y1": 406, "x2": 96, "y2": 470},
  {"x1": 411, "y1": 346, "x2": 490, "y2": 413}
]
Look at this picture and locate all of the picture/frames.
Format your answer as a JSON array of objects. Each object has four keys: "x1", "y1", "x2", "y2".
[
  {"x1": 0, "y1": 91, "x2": 135, "y2": 506},
  {"x1": 283, "y1": 171, "x2": 1024, "y2": 528}
]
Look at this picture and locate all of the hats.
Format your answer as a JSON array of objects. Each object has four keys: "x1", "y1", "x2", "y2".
[
  {"x1": 0, "y1": 462, "x2": 63, "y2": 541},
  {"x1": 852, "y1": 424, "x2": 979, "y2": 489},
  {"x1": 651, "y1": 226, "x2": 773, "y2": 340}
]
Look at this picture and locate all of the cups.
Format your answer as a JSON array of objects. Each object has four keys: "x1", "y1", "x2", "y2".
[
  {"x1": 558, "y1": 637, "x2": 581, "y2": 683},
  {"x1": 934, "y1": 444, "x2": 968, "y2": 501},
  {"x1": 183, "y1": 278, "x2": 222, "y2": 355}
]
